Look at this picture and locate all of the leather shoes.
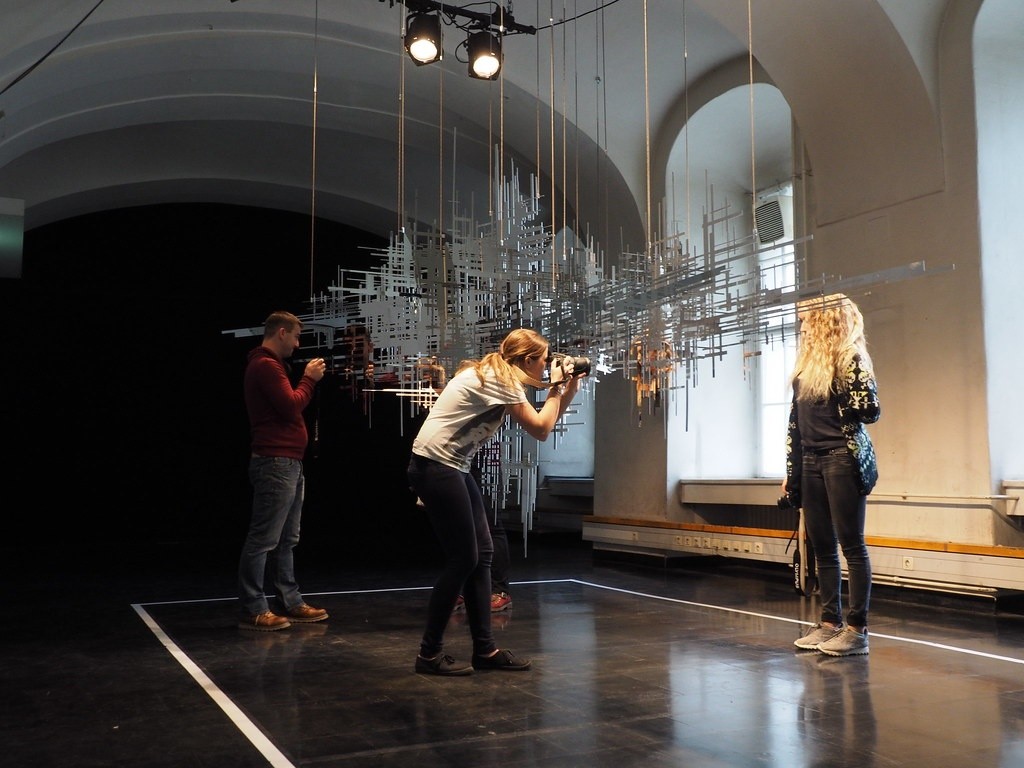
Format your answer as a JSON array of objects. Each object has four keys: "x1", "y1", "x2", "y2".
[
  {"x1": 284, "y1": 603, "x2": 330, "y2": 623},
  {"x1": 237, "y1": 610, "x2": 292, "y2": 631}
]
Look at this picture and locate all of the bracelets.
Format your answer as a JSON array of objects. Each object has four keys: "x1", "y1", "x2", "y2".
[{"x1": 550, "y1": 386, "x2": 563, "y2": 396}]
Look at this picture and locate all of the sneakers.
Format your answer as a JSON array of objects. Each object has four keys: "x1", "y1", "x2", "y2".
[
  {"x1": 793, "y1": 621, "x2": 848, "y2": 650},
  {"x1": 471, "y1": 649, "x2": 532, "y2": 672},
  {"x1": 415, "y1": 652, "x2": 473, "y2": 675},
  {"x1": 816, "y1": 625, "x2": 870, "y2": 657},
  {"x1": 453, "y1": 593, "x2": 467, "y2": 612},
  {"x1": 490, "y1": 592, "x2": 513, "y2": 612}
]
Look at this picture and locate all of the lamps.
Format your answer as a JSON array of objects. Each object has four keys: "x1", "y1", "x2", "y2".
[
  {"x1": 404, "y1": 14, "x2": 445, "y2": 67},
  {"x1": 468, "y1": 31, "x2": 502, "y2": 81}
]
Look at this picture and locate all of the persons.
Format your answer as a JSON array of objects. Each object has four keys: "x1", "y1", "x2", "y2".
[
  {"x1": 430, "y1": 359, "x2": 513, "y2": 611},
  {"x1": 408, "y1": 328, "x2": 586, "y2": 675},
  {"x1": 782, "y1": 293, "x2": 881, "y2": 656},
  {"x1": 236, "y1": 312, "x2": 329, "y2": 630}
]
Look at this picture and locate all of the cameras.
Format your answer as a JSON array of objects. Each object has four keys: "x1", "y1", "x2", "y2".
[
  {"x1": 546, "y1": 353, "x2": 591, "y2": 379},
  {"x1": 777, "y1": 490, "x2": 803, "y2": 510}
]
